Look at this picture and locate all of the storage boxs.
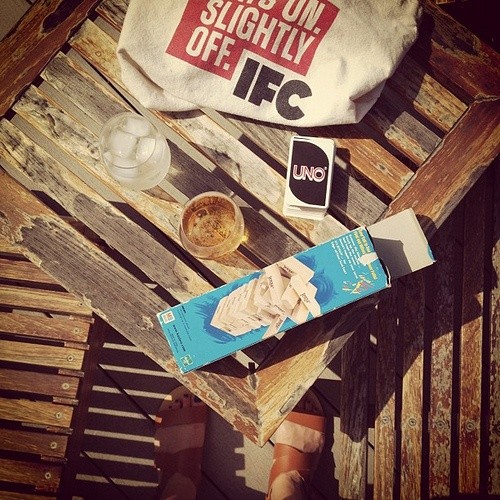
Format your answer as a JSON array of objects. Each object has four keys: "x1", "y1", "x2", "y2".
[{"x1": 149, "y1": 202, "x2": 439, "y2": 374}]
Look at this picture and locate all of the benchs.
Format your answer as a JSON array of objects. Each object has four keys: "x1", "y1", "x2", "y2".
[
  {"x1": 333, "y1": 153, "x2": 500, "y2": 500},
  {"x1": 0, "y1": 214, "x2": 117, "y2": 500}
]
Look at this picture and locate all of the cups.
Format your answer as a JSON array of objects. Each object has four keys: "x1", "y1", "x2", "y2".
[
  {"x1": 99, "y1": 113, "x2": 172, "y2": 191},
  {"x1": 179, "y1": 190, "x2": 245, "y2": 260}
]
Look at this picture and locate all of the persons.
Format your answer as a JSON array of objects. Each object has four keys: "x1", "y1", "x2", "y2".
[{"x1": 153, "y1": 383, "x2": 326, "y2": 499}]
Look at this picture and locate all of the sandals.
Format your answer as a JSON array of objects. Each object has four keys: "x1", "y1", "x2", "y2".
[{"x1": 156, "y1": 386, "x2": 322, "y2": 500}]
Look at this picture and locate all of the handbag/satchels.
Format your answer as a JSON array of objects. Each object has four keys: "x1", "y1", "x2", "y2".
[{"x1": 117, "y1": 0, "x2": 422, "y2": 128}]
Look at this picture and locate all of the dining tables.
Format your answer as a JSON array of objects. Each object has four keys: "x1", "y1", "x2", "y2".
[{"x1": 0, "y1": 0, "x2": 498, "y2": 448}]
